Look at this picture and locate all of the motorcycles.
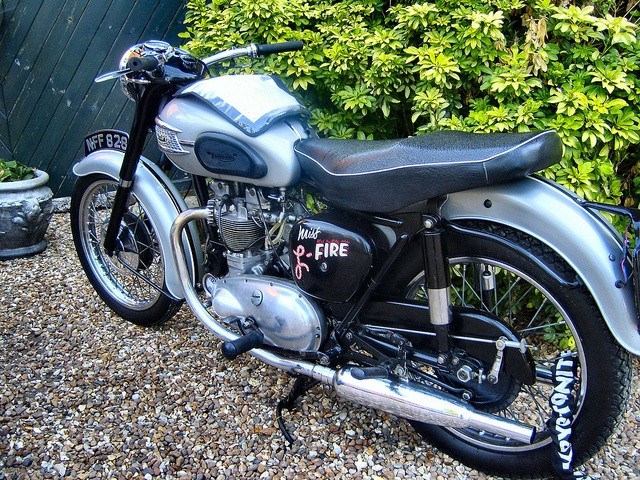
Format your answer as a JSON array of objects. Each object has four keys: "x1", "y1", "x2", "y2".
[{"x1": 70, "y1": 39, "x2": 640, "y2": 479}]
